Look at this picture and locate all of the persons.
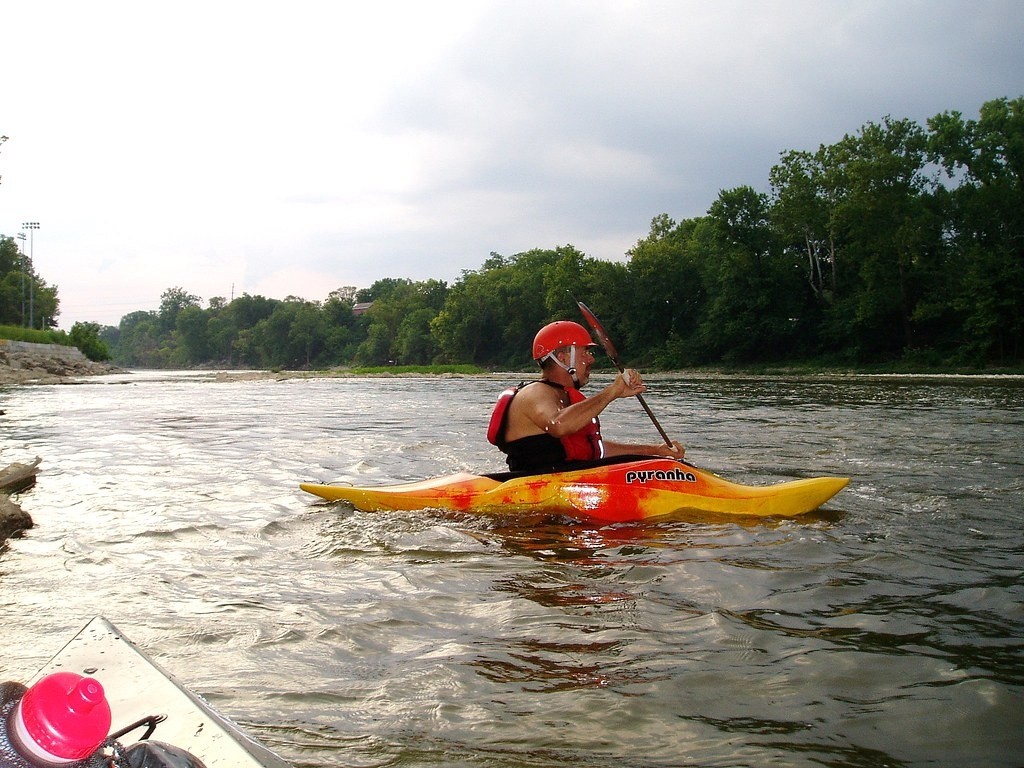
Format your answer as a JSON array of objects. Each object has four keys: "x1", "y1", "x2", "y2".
[{"x1": 503, "y1": 321, "x2": 685, "y2": 473}]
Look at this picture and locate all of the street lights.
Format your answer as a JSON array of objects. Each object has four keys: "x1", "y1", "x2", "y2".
[
  {"x1": 16, "y1": 232, "x2": 26, "y2": 327},
  {"x1": 22, "y1": 221, "x2": 41, "y2": 329}
]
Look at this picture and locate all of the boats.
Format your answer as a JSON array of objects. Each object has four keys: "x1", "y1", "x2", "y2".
[{"x1": 297, "y1": 458, "x2": 849, "y2": 530}]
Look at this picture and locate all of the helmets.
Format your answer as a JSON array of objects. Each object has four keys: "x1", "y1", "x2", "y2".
[{"x1": 532, "y1": 321, "x2": 599, "y2": 361}]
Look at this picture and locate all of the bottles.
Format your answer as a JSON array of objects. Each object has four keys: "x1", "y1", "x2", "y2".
[{"x1": 15, "y1": 671, "x2": 111, "y2": 762}]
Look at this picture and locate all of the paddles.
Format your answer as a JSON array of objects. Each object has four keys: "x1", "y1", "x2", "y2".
[{"x1": 576, "y1": 300, "x2": 685, "y2": 464}]
